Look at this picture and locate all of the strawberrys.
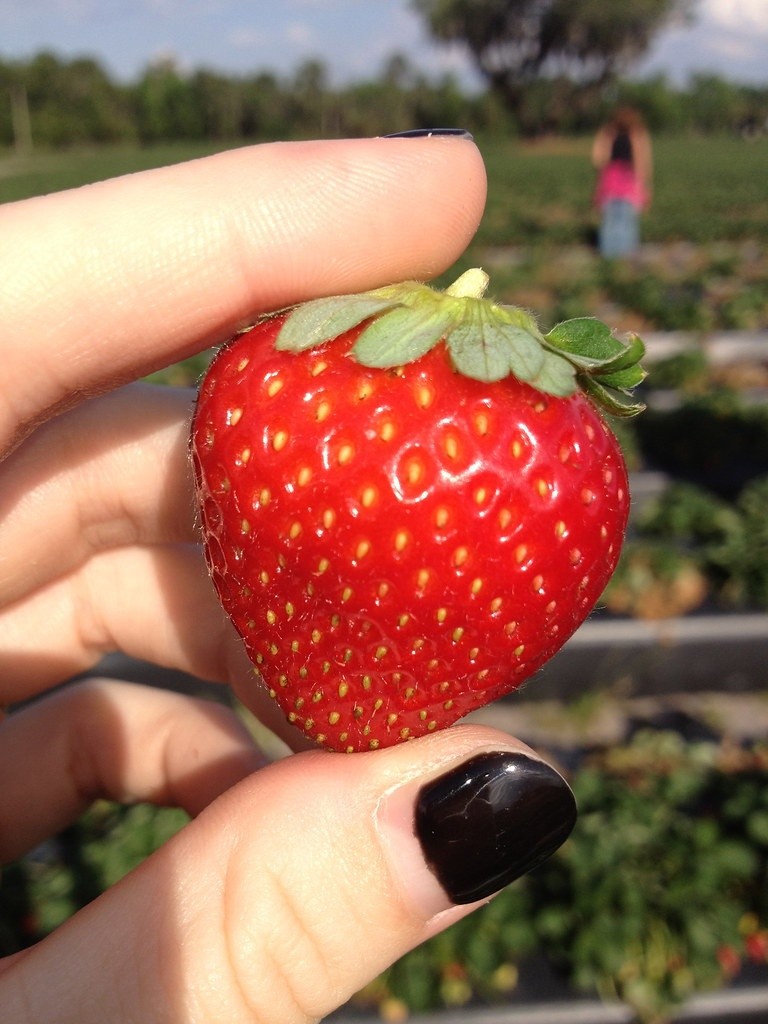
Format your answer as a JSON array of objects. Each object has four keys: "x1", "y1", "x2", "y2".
[{"x1": 188, "y1": 268, "x2": 648, "y2": 755}]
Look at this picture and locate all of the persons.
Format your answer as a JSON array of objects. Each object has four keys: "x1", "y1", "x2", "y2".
[
  {"x1": 0, "y1": 129, "x2": 576, "y2": 1024},
  {"x1": 590, "y1": 95, "x2": 652, "y2": 261}
]
